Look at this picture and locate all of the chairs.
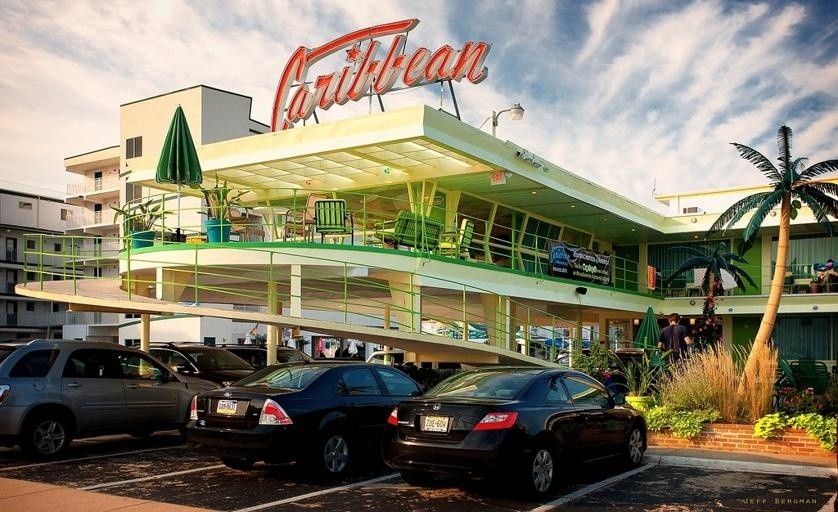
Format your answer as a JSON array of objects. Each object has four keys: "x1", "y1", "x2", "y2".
[
  {"x1": 282, "y1": 193, "x2": 476, "y2": 259},
  {"x1": 772, "y1": 353, "x2": 838, "y2": 410},
  {"x1": 648, "y1": 275, "x2": 685, "y2": 296},
  {"x1": 783, "y1": 263, "x2": 838, "y2": 294}
]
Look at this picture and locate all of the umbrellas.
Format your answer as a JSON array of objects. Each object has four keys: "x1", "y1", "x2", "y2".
[
  {"x1": 155, "y1": 103, "x2": 204, "y2": 241},
  {"x1": 632, "y1": 305, "x2": 663, "y2": 365}
]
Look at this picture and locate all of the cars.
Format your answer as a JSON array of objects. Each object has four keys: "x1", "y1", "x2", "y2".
[{"x1": 1, "y1": 337, "x2": 656, "y2": 503}]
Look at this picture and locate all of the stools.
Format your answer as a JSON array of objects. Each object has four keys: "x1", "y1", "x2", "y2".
[{"x1": 686, "y1": 283, "x2": 701, "y2": 296}]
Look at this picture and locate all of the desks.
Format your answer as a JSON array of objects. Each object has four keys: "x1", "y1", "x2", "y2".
[{"x1": 253, "y1": 206, "x2": 292, "y2": 241}]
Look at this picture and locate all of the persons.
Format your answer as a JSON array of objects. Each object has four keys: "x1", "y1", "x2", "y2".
[{"x1": 657, "y1": 313, "x2": 693, "y2": 366}]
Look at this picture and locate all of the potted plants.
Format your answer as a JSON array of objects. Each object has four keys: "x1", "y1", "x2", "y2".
[
  {"x1": 109, "y1": 197, "x2": 176, "y2": 250},
  {"x1": 197, "y1": 171, "x2": 250, "y2": 244}
]
